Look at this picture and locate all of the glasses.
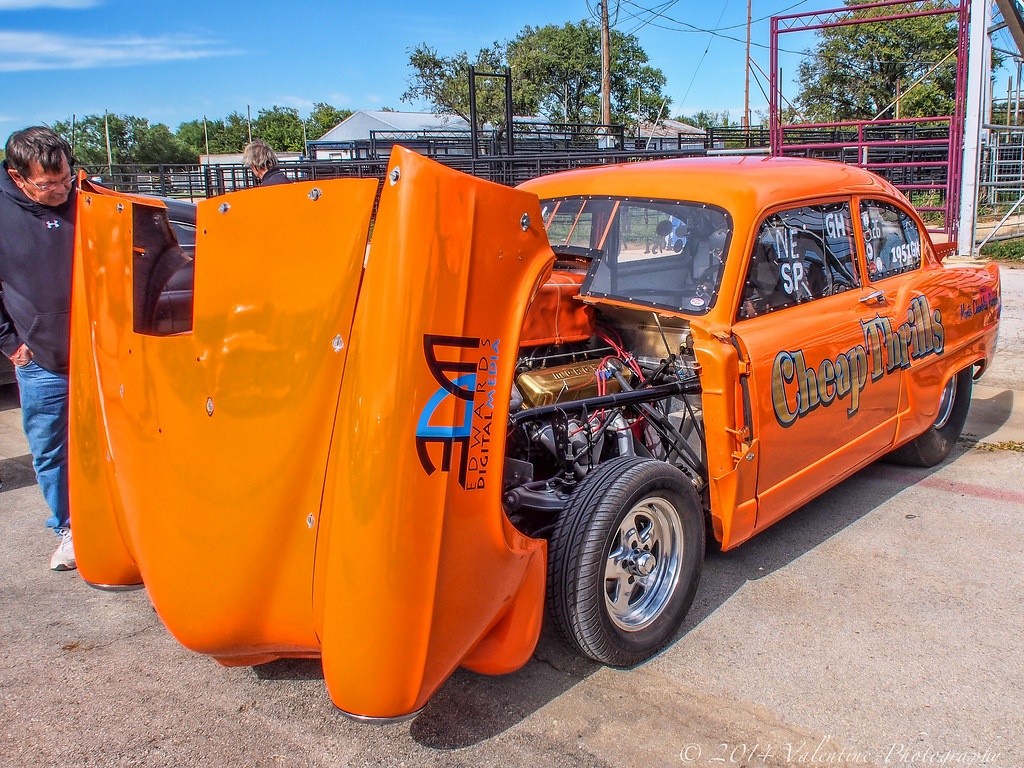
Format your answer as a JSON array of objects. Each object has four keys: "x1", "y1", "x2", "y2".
[{"x1": 26, "y1": 167, "x2": 78, "y2": 192}]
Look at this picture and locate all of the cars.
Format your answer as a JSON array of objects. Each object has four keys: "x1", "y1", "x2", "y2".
[
  {"x1": 0, "y1": 196, "x2": 197, "y2": 387},
  {"x1": 65, "y1": 142, "x2": 1004, "y2": 728}
]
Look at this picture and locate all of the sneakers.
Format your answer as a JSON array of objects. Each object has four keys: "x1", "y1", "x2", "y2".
[{"x1": 50, "y1": 526, "x2": 77, "y2": 571}]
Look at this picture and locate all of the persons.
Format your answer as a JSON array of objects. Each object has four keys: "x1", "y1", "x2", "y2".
[
  {"x1": 242, "y1": 140, "x2": 292, "y2": 185},
  {"x1": 0, "y1": 124, "x2": 81, "y2": 569}
]
